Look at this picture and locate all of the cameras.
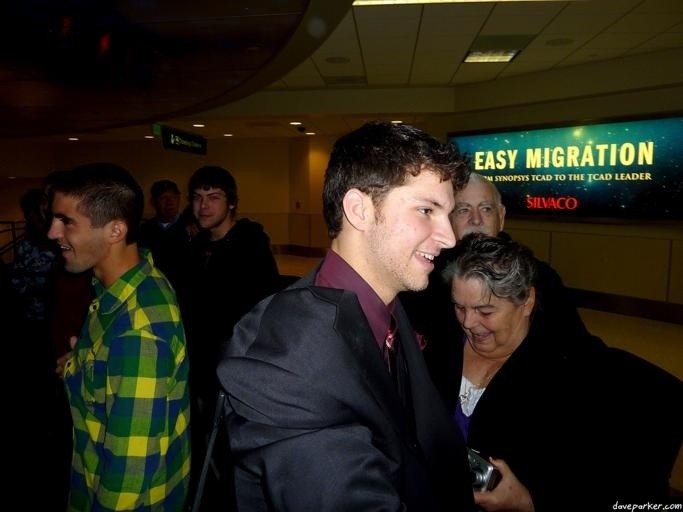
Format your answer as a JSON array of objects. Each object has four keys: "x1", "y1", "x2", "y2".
[{"x1": 466, "y1": 444, "x2": 501, "y2": 495}]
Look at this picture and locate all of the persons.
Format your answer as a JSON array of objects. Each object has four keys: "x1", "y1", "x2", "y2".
[
  {"x1": 214, "y1": 120, "x2": 473, "y2": 512},
  {"x1": 398, "y1": 171, "x2": 581, "y2": 342},
  {"x1": 432, "y1": 233, "x2": 609, "y2": 512}
]
